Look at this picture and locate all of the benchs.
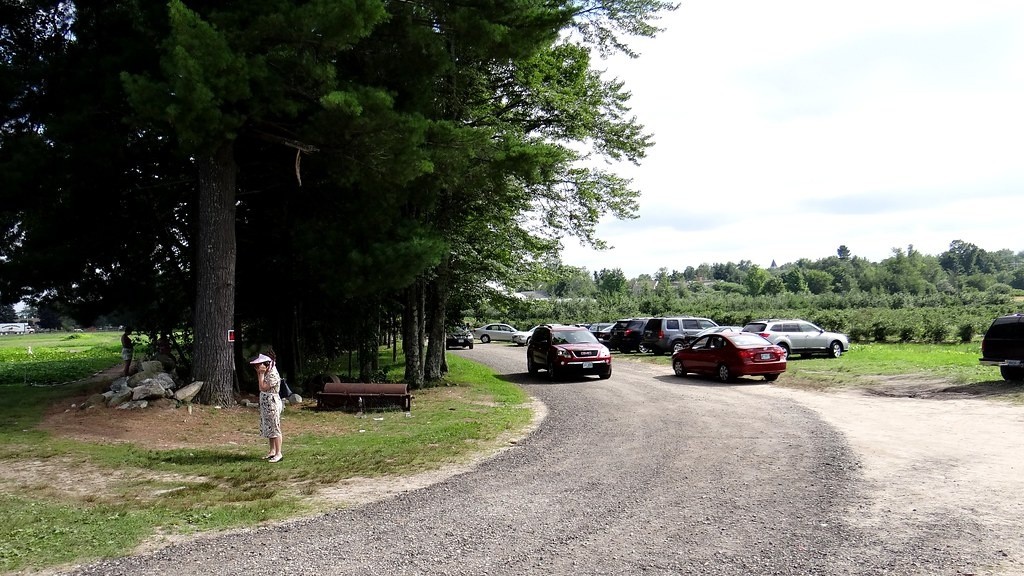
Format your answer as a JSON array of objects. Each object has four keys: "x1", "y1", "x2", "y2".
[{"x1": 314, "y1": 383, "x2": 410, "y2": 413}]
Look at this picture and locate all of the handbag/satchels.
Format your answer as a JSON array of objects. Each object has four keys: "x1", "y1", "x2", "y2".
[{"x1": 279, "y1": 378, "x2": 292, "y2": 399}]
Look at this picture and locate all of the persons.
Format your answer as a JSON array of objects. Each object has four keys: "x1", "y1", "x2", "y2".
[
  {"x1": 150, "y1": 331, "x2": 171, "y2": 354},
  {"x1": 182, "y1": 330, "x2": 188, "y2": 343},
  {"x1": 250, "y1": 347, "x2": 283, "y2": 463},
  {"x1": 121, "y1": 327, "x2": 133, "y2": 376}
]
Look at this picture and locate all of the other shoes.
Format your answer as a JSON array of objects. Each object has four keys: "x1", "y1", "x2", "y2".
[
  {"x1": 268, "y1": 456, "x2": 283, "y2": 463},
  {"x1": 261, "y1": 453, "x2": 276, "y2": 459}
]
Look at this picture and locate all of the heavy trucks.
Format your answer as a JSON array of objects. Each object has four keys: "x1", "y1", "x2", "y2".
[{"x1": 0, "y1": 323, "x2": 35, "y2": 334}]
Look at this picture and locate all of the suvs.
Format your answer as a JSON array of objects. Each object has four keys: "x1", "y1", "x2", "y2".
[
  {"x1": 741, "y1": 319, "x2": 849, "y2": 359},
  {"x1": 527, "y1": 324, "x2": 613, "y2": 383},
  {"x1": 642, "y1": 316, "x2": 719, "y2": 355},
  {"x1": 610, "y1": 317, "x2": 656, "y2": 354}
]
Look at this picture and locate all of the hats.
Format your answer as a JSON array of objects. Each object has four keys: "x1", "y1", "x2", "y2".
[{"x1": 249, "y1": 353, "x2": 273, "y2": 372}]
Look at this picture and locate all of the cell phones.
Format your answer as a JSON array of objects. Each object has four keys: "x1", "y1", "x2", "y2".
[{"x1": 262, "y1": 362, "x2": 270, "y2": 368}]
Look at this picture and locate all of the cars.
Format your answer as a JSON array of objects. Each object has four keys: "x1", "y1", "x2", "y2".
[
  {"x1": 671, "y1": 332, "x2": 787, "y2": 383},
  {"x1": 511, "y1": 323, "x2": 564, "y2": 345},
  {"x1": 978, "y1": 314, "x2": 1024, "y2": 381},
  {"x1": 687, "y1": 326, "x2": 744, "y2": 346},
  {"x1": 568, "y1": 323, "x2": 618, "y2": 351},
  {"x1": 478, "y1": 323, "x2": 542, "y2": 346},
  {"x1": 446, "y1": 322, "x2": 474, "y2": 349}
]
